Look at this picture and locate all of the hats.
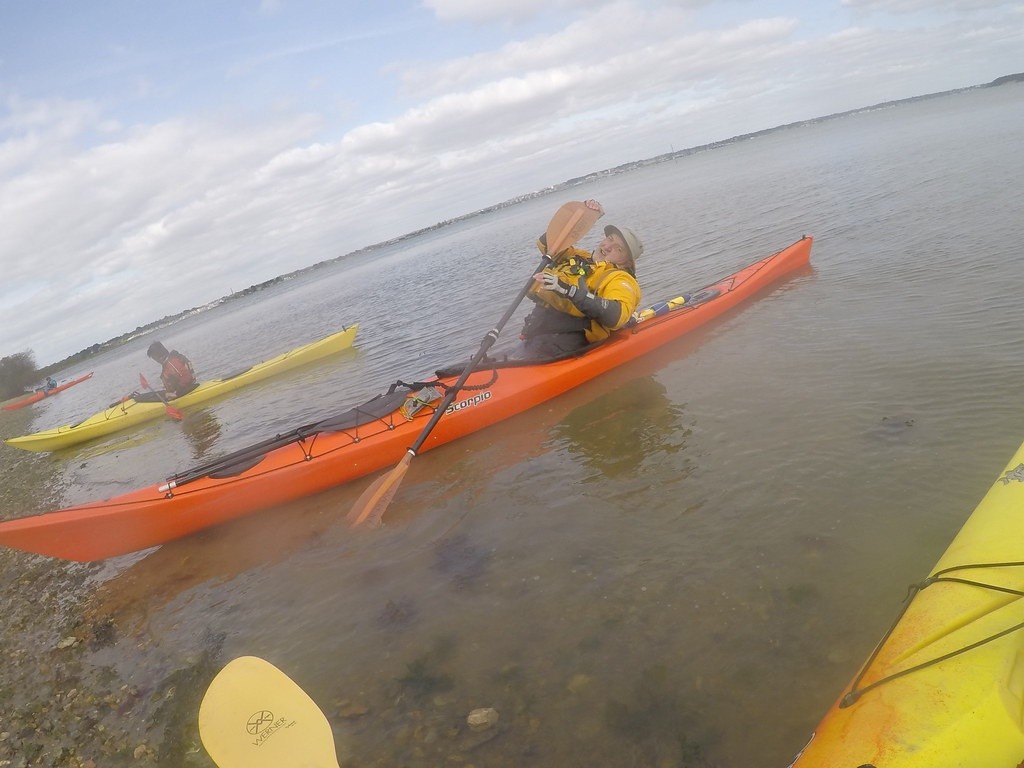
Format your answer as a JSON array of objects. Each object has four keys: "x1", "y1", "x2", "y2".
[{"x1": 604, "y1": 225, "x2": 643, "y2": 274}]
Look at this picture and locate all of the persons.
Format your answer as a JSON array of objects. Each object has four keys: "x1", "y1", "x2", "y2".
[
  {"x1": 147, "y1": 341, "x2": 196, "y2": 401},
  {"x1": 497, "y1": 224, "x2": 644, "y2": 368},
  {"x1": 46, "y1": 377, "x2": 57, "y2": 389}
]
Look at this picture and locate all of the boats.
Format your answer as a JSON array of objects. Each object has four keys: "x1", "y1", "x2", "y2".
[
  {"x1": 789, "y1": 440, "x2": 1024, "y2": 768},
  {"x1": 0, "y1": 235, "x2": 813, "y2": 561},
  {"x1": 3, "y1": 323, "x2": 361, "y2": 451},
  {"x1": 3, "y1": 371, "x2": 94, "y2": 410}
]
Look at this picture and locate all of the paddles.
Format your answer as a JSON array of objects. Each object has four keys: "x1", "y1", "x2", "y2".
[
  {"x1": 196, "y1": 654, "x2": 343, "y2": 768},
  {"x1": 138, "y1": 370, "x2": 183, "y2": 421},
  {"x1": 347, "y1": 200, "x2": 605, "y2": 529}
]
